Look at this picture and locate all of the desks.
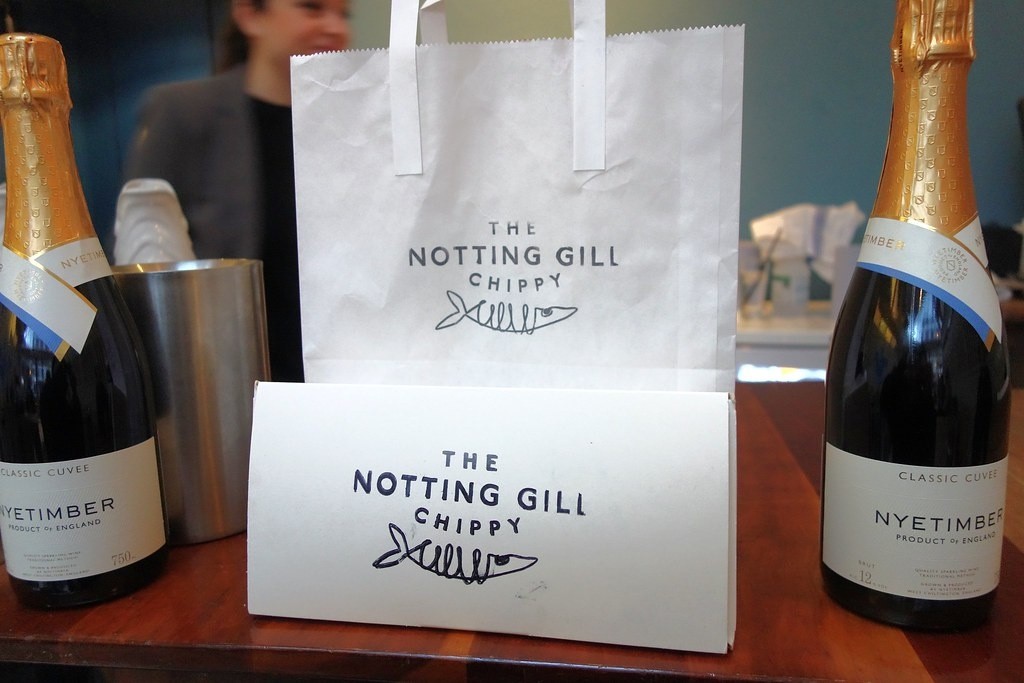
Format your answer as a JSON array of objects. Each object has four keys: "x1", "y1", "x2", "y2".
[{"x1": 0, "y1": 381, "x2": 1024, "y2": 683}]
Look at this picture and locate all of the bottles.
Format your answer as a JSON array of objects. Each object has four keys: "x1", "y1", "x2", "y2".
[
  {"x1": 0, "y1": 34, "x2": 172, "y2": 610},
  {"x1": 820, "y1": 0, "x2": 1012, "y2": 634}
]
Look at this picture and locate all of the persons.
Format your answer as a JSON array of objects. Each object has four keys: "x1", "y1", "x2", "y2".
[{"x1": 125, "y1": 0, "x2": 354, "y2": 384}]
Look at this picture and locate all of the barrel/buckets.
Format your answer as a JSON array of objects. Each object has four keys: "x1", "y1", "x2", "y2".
[{"x1": 106, "y1": 258, "x2": 271, "y2": 547}]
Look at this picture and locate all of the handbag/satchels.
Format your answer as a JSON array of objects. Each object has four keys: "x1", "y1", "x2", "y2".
[{"x1": 289, "y1": 0, "x2": 746, "y2": 399}]
